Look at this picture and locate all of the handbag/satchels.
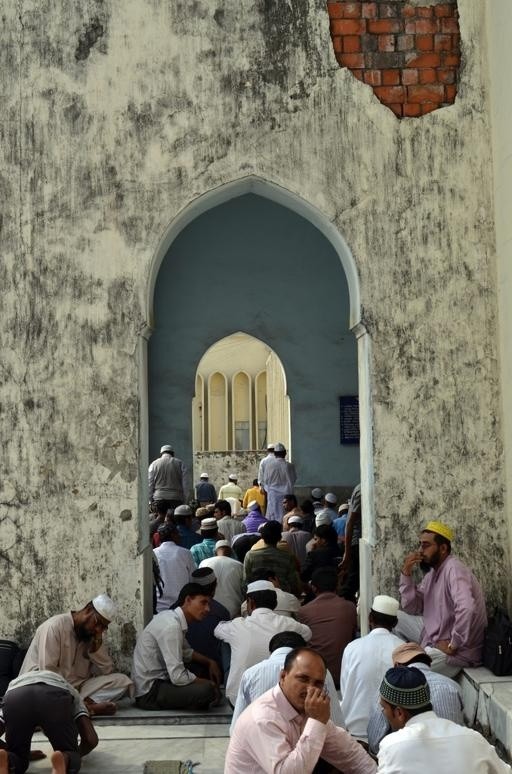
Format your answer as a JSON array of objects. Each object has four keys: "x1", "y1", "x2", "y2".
[{"x1": 482, "y1": 607, "x2": 511, "y2": 676}]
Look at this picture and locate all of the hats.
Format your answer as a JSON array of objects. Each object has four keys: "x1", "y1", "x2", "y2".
[
  {"x1": 161, "y1": 445, "x2": 174, "y2": 453},
  {"x1": 372, "y1": 596, "x2": 399, "y2": 616},
  {"x1": 174, "y1": 504, "x2": 218, "y2": 530},
  {"x1": 192, "y1": 567, "x2": 215, "y2": 585},
  {"x1": 246, "y1": 580, "x2": 275, "y2": 594},
  {"x1": 392, "y1": 641, "x2": 432, "y2": 664},
  {"x1": 93, "y1": 595, "x2": 115, "y2": 622},
  {"x1": 248, "y1": 501, "x2": 258, "y2": 510},
  {"x1": 229, "y1": 474, "x2": 237, "y2": 479},
  {"x1": 312, "y1": 488, "x2": 348, "y2": 512},
  {"x1": 381, "y1": 666, "x2": 431, "y2": 709},
  {"x1": 268, "y1": 443, "x2": 284, "y2": 452},
  {"x1": 424, "y1": 521, "x2": 453, "y2": 541},
  {"x1": 200, "y1": 473, "x2": 208, "y2": 478},
  {"x1": 288, "y1": 517, "x2": 302, "y2": 523}
]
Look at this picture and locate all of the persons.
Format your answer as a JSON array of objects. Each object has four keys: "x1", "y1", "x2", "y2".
[
  {"x1": 17, "y1": 593, "x2": 132, "y2": 716},
  {"x1": 391, "y1": 521, "x2": 486, "y2": 678},
  {"x1": 131, "y1": 442, "x2": 360, "y2": 710},
  {"x1": 224, "y1": 596, "x2": 511, "y2": 774},
  {"x1": 0, "y1": 670, "x2": 98, "y2": 773}
]
